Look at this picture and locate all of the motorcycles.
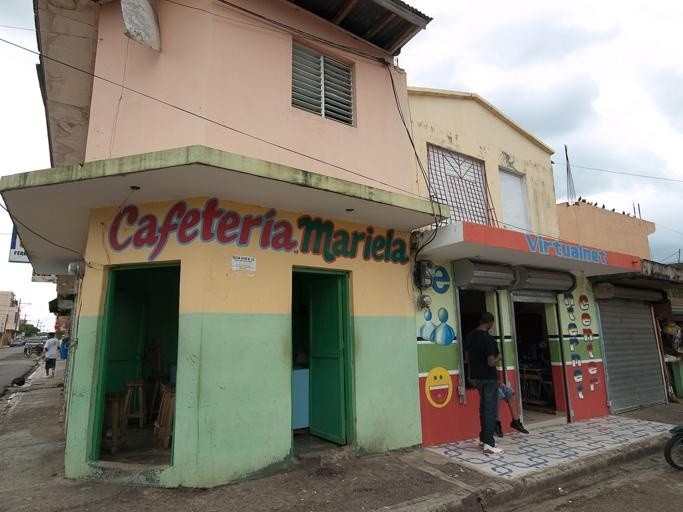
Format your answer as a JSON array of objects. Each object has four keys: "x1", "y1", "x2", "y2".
[{"x1": 663, "y1": 422, "x2": 683, "y2": 473}]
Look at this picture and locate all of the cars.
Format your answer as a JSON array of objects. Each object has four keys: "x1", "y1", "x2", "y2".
[
  {"x1": 9, "y1": 337, "x2": 24, "y2": 347},
  {"x1": 23, "y1": 335, "x2": 47, "y2": 356}
]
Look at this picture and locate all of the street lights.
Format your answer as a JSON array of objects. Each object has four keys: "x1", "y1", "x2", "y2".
[{"x1": 13, "y1": 299, "x2": 32, "y2": 342}]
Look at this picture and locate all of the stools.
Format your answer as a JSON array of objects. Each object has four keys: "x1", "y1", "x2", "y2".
[
  {"x1": 152, "y1": 382, "x2": 174, "y2": 449},
  {"x1": 103, "y1": 379, "x2": 147, "y2": 449}
]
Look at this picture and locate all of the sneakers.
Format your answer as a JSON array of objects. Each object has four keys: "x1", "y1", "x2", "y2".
[
  {"x1": 479, "y1": 442, "x2": 504, "y2": 456},
  {"x1": 511, "y1": 418, "x2": 529, "y2": 434},
  {"x1": 495, "y1": 421, "x2": 503, "y2": 437}
]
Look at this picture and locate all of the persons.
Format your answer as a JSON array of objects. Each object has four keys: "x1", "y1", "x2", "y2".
[
  {"x1": 467, "y1": 312, "x2": 529, "y2": 454},
  {"x1": 44, "y1": 332, "x2": 60, "y2": 379}
]
